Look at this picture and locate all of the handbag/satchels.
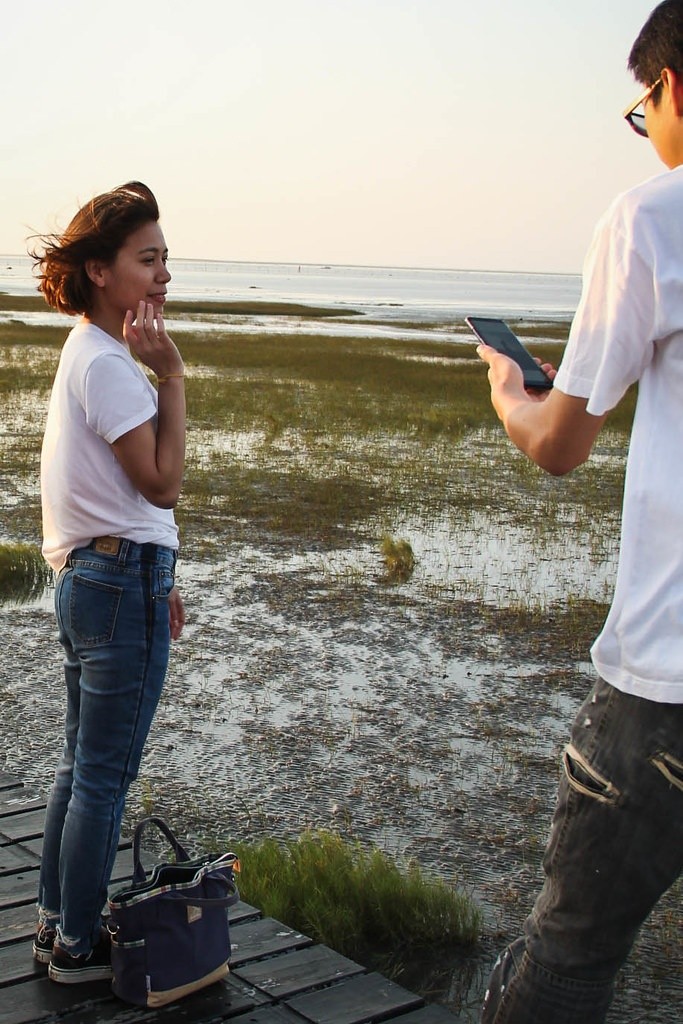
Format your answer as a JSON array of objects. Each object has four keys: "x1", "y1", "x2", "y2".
[{"x1": 99, "y1": 817, "x2": 239, "y2": 1008}]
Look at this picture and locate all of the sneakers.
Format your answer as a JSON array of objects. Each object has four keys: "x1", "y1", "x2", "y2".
[
  {"x1": 33, "y1": 907, "x2": 60, "y2": 963},
  {"x1": 48, "y1": 926, "x2": 113, "y2": 983}
]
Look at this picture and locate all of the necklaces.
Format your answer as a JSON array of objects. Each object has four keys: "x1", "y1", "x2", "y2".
[{"x1": 90, "y1": 321, "x2": 128, "y2": 349}]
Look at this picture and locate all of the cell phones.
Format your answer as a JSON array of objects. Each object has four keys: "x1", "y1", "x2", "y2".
[{"x1": 463, "y1": 315, "x2": 554, "y2": 389}]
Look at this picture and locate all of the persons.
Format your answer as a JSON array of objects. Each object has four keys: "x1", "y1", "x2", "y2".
[
  {"x1": 476, "y1": 1, "x2": 683, "y2": 1024},
  {"x1": 24, "y1": 181, "x2": 186, "y2": 985}
]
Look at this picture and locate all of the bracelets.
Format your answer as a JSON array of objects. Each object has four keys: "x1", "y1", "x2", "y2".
[{"x1": 157, "y1": 374, "x2": 186, "y2": 384}]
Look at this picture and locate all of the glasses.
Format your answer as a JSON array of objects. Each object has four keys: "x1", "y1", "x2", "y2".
[{"x1": 622, "y1": 78, "x2": 661, "y2": 137}]
